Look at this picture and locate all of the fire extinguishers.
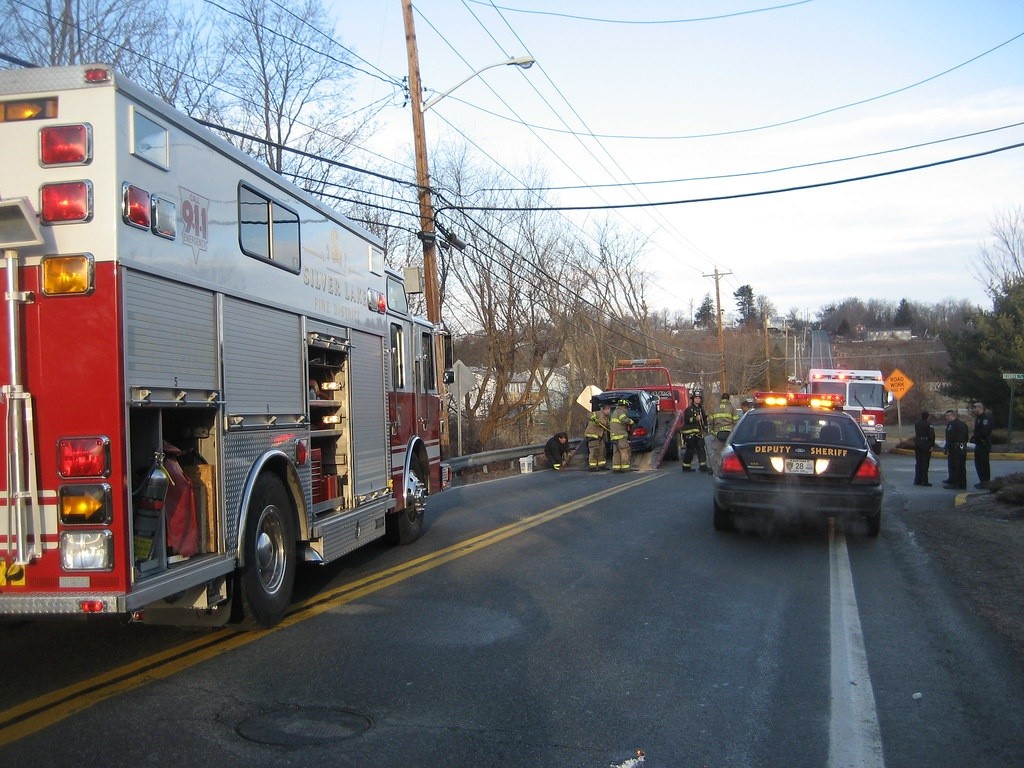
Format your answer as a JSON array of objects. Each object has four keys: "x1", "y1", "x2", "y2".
[{"x1": 134, "y1": 451, "x2": 176, "y2": 564}]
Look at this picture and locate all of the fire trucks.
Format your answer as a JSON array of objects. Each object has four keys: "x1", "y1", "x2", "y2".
[
  {"x1": 801, "y1": 368, "x2": 894, "y2": 459},
  {"x1": 1, "y1": 62, "x2": 456, "y2": 640}
]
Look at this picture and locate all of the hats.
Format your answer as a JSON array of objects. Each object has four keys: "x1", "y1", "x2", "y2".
[
  {"x1": 690, "y1": 389, "x2": 704, "y2": 398},
  {"x1": 613, "y1": 399, "x2": 631, "y2": 407}
]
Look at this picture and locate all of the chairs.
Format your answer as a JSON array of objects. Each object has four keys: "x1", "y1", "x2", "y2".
[
  {"x1": 753, "y1": 420, "x2": 779, "y2": 441},
  {"x1": 816, "y1": 426, "x2": 843, "y2": 444}
]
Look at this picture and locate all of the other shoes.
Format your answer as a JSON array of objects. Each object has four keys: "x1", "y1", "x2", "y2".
[
  {"x1": 614, "y1": 468, "x2": 631, "y2": 473},
  {"x1": 942, "y1": 480, "x2": 950, "y2": 483},
  {"x1": 591, "y1": 465, "x2": 610, "y2": 472},
  {"x1": 975, "y1": 483, "x2": 987, "y2": 489},
  {"x1": 914, "y1": 481, "x2": 932, "y2": 486},
  {"x1": 682, "y1": 465, "x2": 697, "y2": 472},
  {"x1": 699, "y1": 466, "x2": 711, "y2": 471},
  {"x1": 943, "y1": 485, "x2": 961, "y2": 489}
]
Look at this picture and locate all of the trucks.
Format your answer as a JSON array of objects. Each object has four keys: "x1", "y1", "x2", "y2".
[{"x1": 606, "y1": 357, "x2": 690, "y2": 465}]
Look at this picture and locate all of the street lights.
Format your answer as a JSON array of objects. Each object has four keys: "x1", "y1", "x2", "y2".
[{"x1": 400, "y1": 1, "x2": 534, "y2": 492}]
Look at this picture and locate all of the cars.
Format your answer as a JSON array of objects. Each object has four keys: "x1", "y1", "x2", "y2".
[
  {"x1": 590, "y1": 390, "x2": 660, "y2": 453},
  {"x1": 713, "y1": 392, "x2": 886, "y2": 538}
]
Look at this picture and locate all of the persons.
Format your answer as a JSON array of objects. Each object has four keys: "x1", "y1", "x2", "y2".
[
  {"x1": 544, "y1": 433, "x2": 573, "y2": 470},
  {"x1": 710, "y1": 392, "x2": 738, "y2": 463},
  {"x1": 739, "y1": 401, "x2": 751, "y2": 414},
  {"x1": 943, "y1": 411, "x2": 968, "y2": 492},
  {"x1": 912, "y1": 411, "x2": 936, "y2": 486},
  {"x1": 969, "y1": 401, "x2": 991, "y2": 489},
  {"x1": 679, "y1": 390, "x2": 712, "y2": 471},
  {"x1": 940, "y1": 410, "x2": 957, "y2": 483},
  {"x1": 584, "y1": 403, "x2": 612, "y2": 471},
  {"x1": 609, "y1": 399, "x2": 637, "y2": 472}
]
